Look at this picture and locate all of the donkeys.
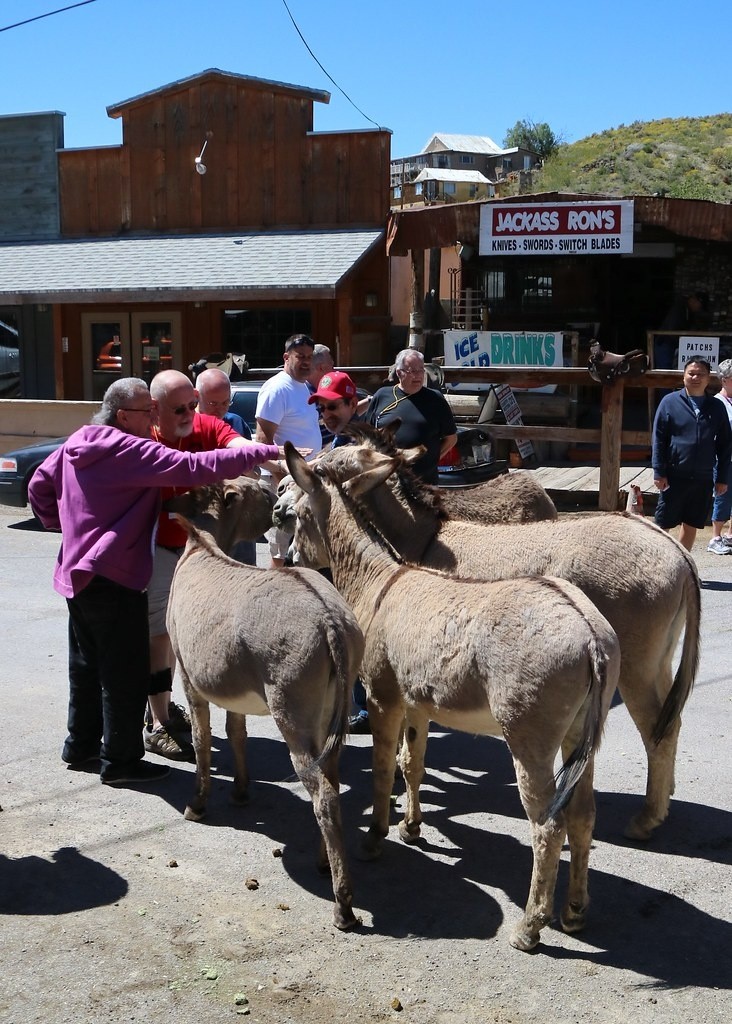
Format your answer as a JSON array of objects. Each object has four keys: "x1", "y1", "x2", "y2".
[{"x1": 163, "y1": 417, "x2": 703, "y2": 953}]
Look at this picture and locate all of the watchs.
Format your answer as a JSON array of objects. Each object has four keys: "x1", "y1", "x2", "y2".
[{"x1": 252, "y1": 465, "x2": 261, "y2": 479}]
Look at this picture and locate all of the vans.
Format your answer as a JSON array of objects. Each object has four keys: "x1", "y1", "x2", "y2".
[{"x1": 0, "y1": 320, "x2": 20, "y2": 378}]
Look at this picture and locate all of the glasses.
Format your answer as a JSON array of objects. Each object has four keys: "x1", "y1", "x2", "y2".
[
  {"x1": 174, "y1": 400, "x2": 199, "y2": 415},
  {"x1": 400, "y1": 368, "x2": 426, "y2": 374},
  {"x1": 208, "y1": 401, "x2": 233, "y2": 407},
  {"x1": 124, "y1": 402, "x2": 159, "y2": 417},
  {"x1": 315, "y1": 401, "x2": 345, "y2": 413}
]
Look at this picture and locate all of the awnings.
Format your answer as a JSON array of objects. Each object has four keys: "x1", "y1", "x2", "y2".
[{"x1": 0, "y1": 231, "x2": 384, "y2": 305}]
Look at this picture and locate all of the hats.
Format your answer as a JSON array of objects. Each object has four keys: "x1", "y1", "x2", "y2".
[{"x1": 308, "y1": 371, "x2": 356, "y2": 405}]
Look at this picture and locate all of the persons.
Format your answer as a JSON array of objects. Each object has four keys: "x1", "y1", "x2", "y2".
[
  {"x1": 365, "y1": 348, "x2": 458, "y2": 485},
  {"x1": 195, "y1": 368, "x2": 261, "y2": 566},
  {"x1": 255, "y1": 333, "x2": 400, "y2": 735},
  {"x1": 143, "y1": 369, "x2": 322, "y2": 761},
  {"x1": 27, "y1": 378, "x2": 314, "y2": 783},
  {"x1": 708, "y1": 358, "x2": 732, "y2": 555},
  {"x1": 653, "y1": 291, "x2": 709, "y2": 400},
  {"x1": 652, "y1": 355, "x2": 732, "y2": 552}
]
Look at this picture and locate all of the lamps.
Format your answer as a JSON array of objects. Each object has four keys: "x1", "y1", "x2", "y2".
[
  {"x1": 194, "y1": 128, "x2": 214, "y2": 174},
  {"x1": 364, "y1": 289, "x2": 379, "y2": 307},
  {"x1": 194, "y1": 301, "x2": 207, "y2": 308},
  {"x1": 36, "y1": 303, "x2": 48, "y2": 312}
]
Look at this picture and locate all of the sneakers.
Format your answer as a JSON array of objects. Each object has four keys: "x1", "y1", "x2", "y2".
[
  {"x1": 146, "y1": 702, "x2": 192, "y2": 736},
  {"x1": 144, "y1": 721, "x2": 196, "y2": 760},
  {"x1": 722, "y1": 532, "x2": 732, "y2": 553},
  {"x1": 707, "y1": 536, "x2": 731, "y2": 555},
  {"x1": 100, "y1": 760, "x2": 170, "y2": 784}
]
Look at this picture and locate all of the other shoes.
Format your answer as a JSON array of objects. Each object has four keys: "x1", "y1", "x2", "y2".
[
  {"x1": 62, "y1": 742, "x2": 103, "y2": 764},
  {"x1": 347, "y1": 715, "x2": 373, "y2": 734}
]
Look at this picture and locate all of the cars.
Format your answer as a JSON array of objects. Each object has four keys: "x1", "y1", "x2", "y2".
[
  {"x1": 97, "y1": 339, "x2": 174, "y2": 377},
  {"x1": 0, "y1": 380, "x2": 496, "y2": 523}
]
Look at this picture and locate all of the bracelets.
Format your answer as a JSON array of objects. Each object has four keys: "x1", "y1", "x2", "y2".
[
  {"x1": 367, "y1": 395, "x2": 371, "y2": 403},
  {"x1": 271, "y1": 460, "x2": 281, "y2": 473}
]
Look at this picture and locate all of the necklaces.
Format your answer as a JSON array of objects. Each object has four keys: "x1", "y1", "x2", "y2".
[{"x1": 374, "y1": 383, "x2": 412, "y2": 429}]
